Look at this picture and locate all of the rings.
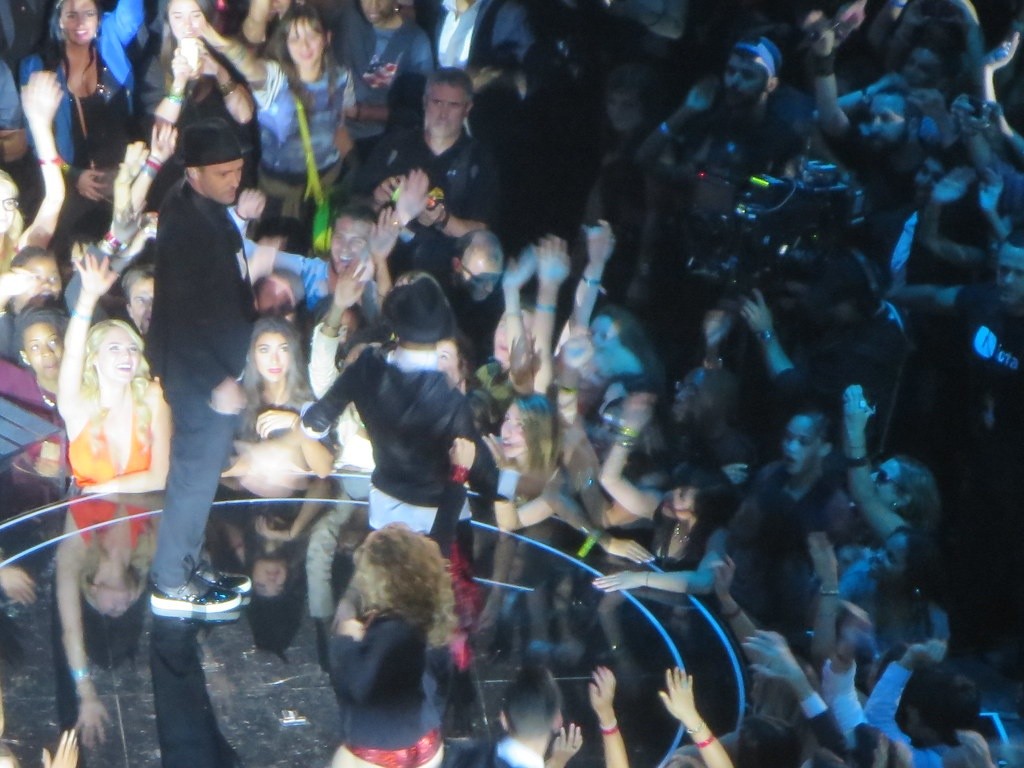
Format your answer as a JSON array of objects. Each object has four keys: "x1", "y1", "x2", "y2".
[{"x1": 392, "y1": 221, "x2": 400, "y2": 227}]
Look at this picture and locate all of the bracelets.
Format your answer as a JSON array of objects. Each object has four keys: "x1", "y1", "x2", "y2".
[
  {"x1": 166, "y1": 90, "x2": 186, "y2": 103},
  {"x1": 323, "y1": 317, "x2": 339, "y2": 332},
  {"x1": 71, "y1": 665, "x2": 91, "y2": 678},
  {"x1": 695, "y1": 736, "x2": 715, "y2": 748},
  {"x1": 723, "y1": 603, "x2": 743, "y2": 618},
  {"x1": 616, "y1": 426, "x2": 639, "y2": 439},
  {"x1": 599, "y1": 724, "x2": 620, "y2": 734},
  {"x1": 225, "y1": 42, "x2": 247, "y2": 63},
  {"x1": 536, "y1": 302, "x2": 557, "y2": 313},
  {"x1": 218, "y1": 76, "x2": 238, "y2": 97},
  {"x1": 891, "y1": 0, "x2": 905, "y2": 9},
  {"x1": 688, "y1": 718, "x2": 707, "y2": 734},
  {"x1": 142, "y1": 166, "x2": 158, "y2": 179},
  {"x1": 645, "y1": 571, "x2": 651, "y2": 586},
  {"x1": 846, "y1": 455, "x2": 870, "y2": 469},
  {"x1": 71, "y1": 308, "x2": 93, "y2": 322},
  {"x1": 99, "y1": 232, "x2": 123, "y2": 253},
  {"x1": 815, "y1": 54, "x2": 836, "y2": 77},
  {"x1": 39, "y1": 155, "x2": 63, "y2": 165},
  {"x1": 146, "y1": 159, "x2": 161, "y2": 170},
  {"x1": 819, "y1": 585, "x2": 840, "y2": 597},
  {"x1": 613, "y1": 438, "x2": 637, "y2": 449},
  {"x1": 582, "y1": 273, "x2": 602, "y2": 286},
  {"x1": 354, "y1": 106, "x2": 364, "y2": 123},
  {"x1": 598, "y1": 717, "x2": 618, "y2": 730},
  {"x1": 506, "y1": 311, "x2": 525, "y2": 321},
  {"x1": 147, "y1": 155, "x2": 163, "y2": 168},
  {"x1": 557, "y1": 385, "x2": 579, "y2": 394},
  {"x1": 754, "y1": 327, "x2": 778, "y2": 344},
  {"x1": 661, "y1": 122, "x2": 676, "y2": 138}
]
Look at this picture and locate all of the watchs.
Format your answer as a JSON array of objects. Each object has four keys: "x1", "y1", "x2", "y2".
[
  {"x1": 860, "y1": 88, "x2": 873, "y2": 105},
  {"x1": 433, "y1": 209, "x2": 450, "y2": 231}
]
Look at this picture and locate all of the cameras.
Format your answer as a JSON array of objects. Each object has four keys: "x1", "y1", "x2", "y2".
[{"x1": 967, "y1": 98, "x2": 991, "y2": 121}]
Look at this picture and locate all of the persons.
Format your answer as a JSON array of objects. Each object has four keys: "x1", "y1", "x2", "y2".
[
  {"x1": 331, "y1": 433, "x2": 478, "y2": 767},
  {"x1": 149, "y1": 117, "x2": 254, "y2": 619},
  {"x1": 305, "y1": 272, "x2": 477, "y2": 556},
  {"x1": 2, "y1": 0, "x2": 1023, "y2": 766}
]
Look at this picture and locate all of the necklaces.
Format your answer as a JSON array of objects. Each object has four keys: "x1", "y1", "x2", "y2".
[
  {"x1": 674, "y1": 523, "x2": 690, "y2": 546},
  {"x1": 36, "y1": 378, "x2": 57, "y2": 409}
]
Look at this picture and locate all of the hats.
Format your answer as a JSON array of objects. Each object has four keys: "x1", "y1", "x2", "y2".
[
  {"x1": 179, "y1": 118, "x2": 257, "y2": 168},
  {"x1": 384, "y1": 277, "x2": 453, "y2": 345}
]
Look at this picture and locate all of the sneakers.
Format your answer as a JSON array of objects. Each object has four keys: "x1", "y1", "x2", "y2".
[{"x1": 151, "y1": 564, "x2": 253, "y2": 618}]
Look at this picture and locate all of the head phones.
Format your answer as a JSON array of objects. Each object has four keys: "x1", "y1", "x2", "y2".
[{"x1": 844, "y1": 246, "x2": 884, "y2": 320}]
